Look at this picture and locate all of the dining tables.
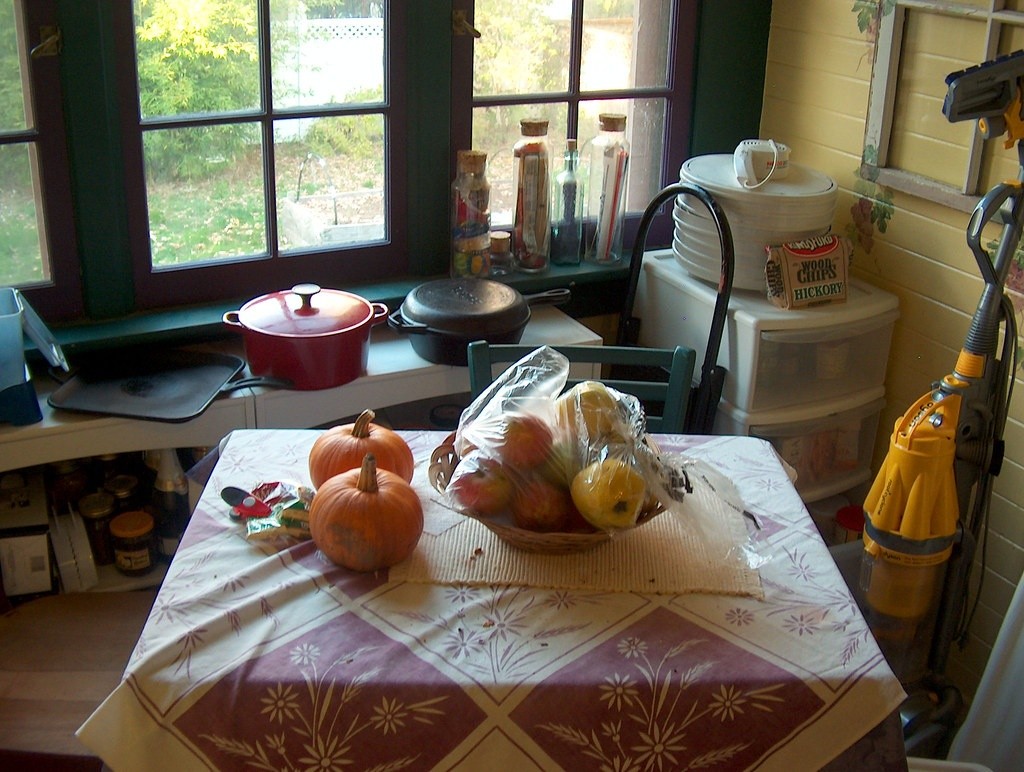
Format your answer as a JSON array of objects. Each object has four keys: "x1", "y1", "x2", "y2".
[{"x1": 74, "y1": 429, "x2": 921, "y2": 772}]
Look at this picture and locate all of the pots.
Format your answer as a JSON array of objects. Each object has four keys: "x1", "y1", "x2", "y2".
[
  {"x1": 222, "y1": 283, "x2": 391, "y2": 390},
  {"x1": 387, "y1": 277, "x2": 571, "y2": 367}
]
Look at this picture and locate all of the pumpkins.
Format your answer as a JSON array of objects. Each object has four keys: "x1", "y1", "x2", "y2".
[
  {"x1": 309, "y1": 452, "x2": 423, "y2": 571},
  {"x1": 308, "y1": 409, "x2": 415, "y2": 486}
]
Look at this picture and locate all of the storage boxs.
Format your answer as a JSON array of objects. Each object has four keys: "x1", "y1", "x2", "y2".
[{"x1": 0, "y1": 472, "x2": 57, "y2": 599}]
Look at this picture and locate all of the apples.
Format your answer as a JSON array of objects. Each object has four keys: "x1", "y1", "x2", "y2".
[{"x1": 451, "y1": 386, "x2": 659, "y2": 533}]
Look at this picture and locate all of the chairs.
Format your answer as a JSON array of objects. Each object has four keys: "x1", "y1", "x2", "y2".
[{"x1": 467, "y1": 340, "x2": 691, "y2": 434}]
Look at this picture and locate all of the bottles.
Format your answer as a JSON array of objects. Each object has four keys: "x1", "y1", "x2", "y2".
[
  {"x1": 449, "y1": 149, "x2": 492, "y2": 280},
  {"x1": 511, "y1": 118, "x2": 554, "y2": 274},
  {"x1": 548, "y1": 138, "x2": 586, "y2": 266},
  {"x1": 57, "y1": 444, "x2": 210, "y2": 580},
  {"x1": 489, "y1": 230, "x2": 515, "y2": 276},
  {"x1": 576, "y1": 112, "x2": 630, "y2": 266}
]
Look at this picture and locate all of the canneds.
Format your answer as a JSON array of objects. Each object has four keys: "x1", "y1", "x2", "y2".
[{"x1": 75, "y1": 476, "x2": 158, "y2": 577}]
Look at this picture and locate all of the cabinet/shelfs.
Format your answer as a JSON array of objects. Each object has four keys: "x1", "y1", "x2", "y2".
[{"x1": 645, "y1": 245, "x2": 901, "y2": 546}]
